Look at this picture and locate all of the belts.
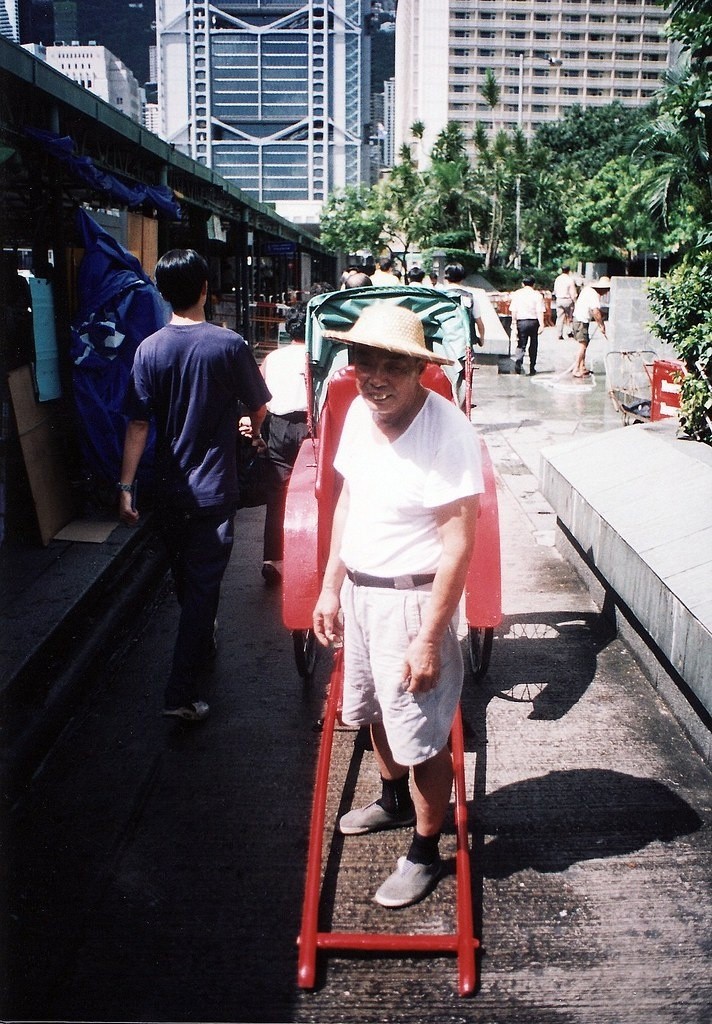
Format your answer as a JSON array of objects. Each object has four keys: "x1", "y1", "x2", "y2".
[{"x1": 345, "y1": 566, "x2": 436, "y2": 590}]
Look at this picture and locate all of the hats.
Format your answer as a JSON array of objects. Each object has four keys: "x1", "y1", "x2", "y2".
[
  {"x1": 592, "y1": 276, "x2": 612, "y2": 288},
  {"x1": 321, "y1": 305, "x2": 456, "y2": 365}
]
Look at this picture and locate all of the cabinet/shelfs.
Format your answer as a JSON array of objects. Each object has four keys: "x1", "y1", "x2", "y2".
[{"x1": 65, "y1": 209, "x2": 160, "y2": 317}]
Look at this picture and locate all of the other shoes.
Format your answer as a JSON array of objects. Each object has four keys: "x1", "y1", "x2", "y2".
[
  {"x1": 339, "y1": 798, "x2": 416, "y2": 834},
  {"x1": 514, "y1": 360, "x2": 523, "y2": 373},
  {"x1": 374, "y1": 856, "x2": 444, "y2": 907},
  {"x1": 262, "y1": 564, "x2": 281, "y2": 583},
  {"x1": 211, "y1": 620, "x2": 219, "y2": 649},
  {"x1": 164, "y1": 701, "x2": 210, "y2": 723},
  {"x1": 531, "y1": 368, "x2": 537, "y2": 376}
]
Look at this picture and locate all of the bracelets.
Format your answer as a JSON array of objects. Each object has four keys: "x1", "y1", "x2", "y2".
[
  {"x1": 117, "y1": 482, "x2": 134, "y2": 491},
  {"x1": 252, "y1": 434, "x2": 262, "y2": 439}
]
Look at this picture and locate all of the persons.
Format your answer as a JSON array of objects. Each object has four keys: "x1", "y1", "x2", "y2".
[
  {"x1": 509, "y1": 275, "x2": 546, "y2": 375},
  {"x1": 553, "y1": 265, "x2": 578, "y2": 339},
  {"x1": 239, "y1": 302, "x2": 307, "y2": 588},
  {"x1": 313, "y1": 304, "x2": 486, "y2": 909},
  {"x1": 118, "y1": 249, "x2": 273, "y2": 726},
  {"x1": 339, "y1": 256, "x2": 485, "y2": 348},
  {"x1": 573, "y1": 277, "x2": 610, "y2": 377}
]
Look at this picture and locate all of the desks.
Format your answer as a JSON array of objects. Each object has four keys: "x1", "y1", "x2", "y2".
[{"x1": 257, "y1": 301, "x2": 276, "y2": 334}]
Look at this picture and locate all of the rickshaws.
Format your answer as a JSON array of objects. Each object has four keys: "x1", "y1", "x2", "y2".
[{"x1": 279, "y1": 280, "x2": 503, "y2": 1003}]
